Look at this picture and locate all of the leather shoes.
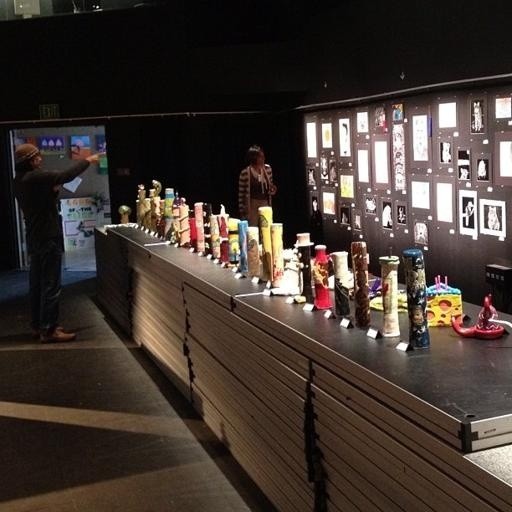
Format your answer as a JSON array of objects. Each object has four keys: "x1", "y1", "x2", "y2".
[
  {"x1": 40, "y1": 330, "x2": 75, "y2": 343},
  {"x1": 32, "y1": 327, "x2": 63, "y2": 338}
]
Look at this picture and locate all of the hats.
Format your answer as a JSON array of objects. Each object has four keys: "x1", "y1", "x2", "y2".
[{"x1": 15, "y1": 144, "x2": 39, "y2": 163}]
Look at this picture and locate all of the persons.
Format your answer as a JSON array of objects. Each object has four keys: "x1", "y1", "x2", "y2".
[
  {"x1": 12, "y1": 143, "x2": 105, "y2": 343},
  {"x1": 238, "y1": 144, "x2": 279, "y2": 226}
]
